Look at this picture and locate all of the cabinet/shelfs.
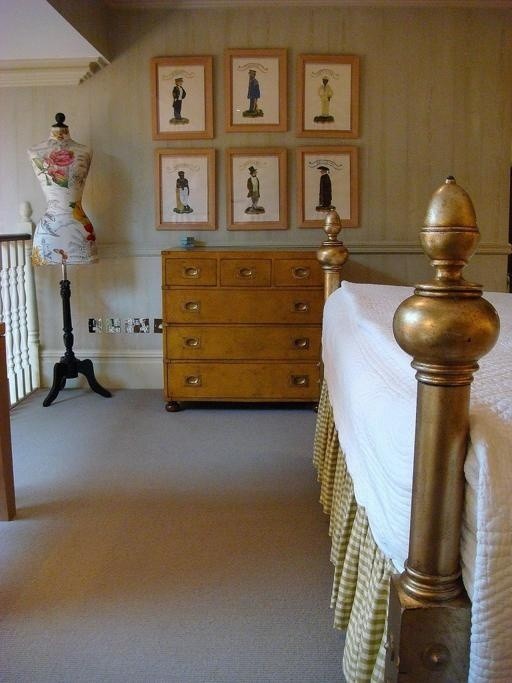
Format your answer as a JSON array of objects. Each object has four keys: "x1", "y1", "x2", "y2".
[{"x1": 159, "y1": 244, "x2": 327, "y2": 412}]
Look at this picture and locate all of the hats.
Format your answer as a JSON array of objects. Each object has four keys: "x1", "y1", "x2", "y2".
[
  {"x1": 317, "y1": 166, "x2": 329, "y2": 170},
  {"x1": 175, "y1": 78, "x2": 184, "y2": 83},
  {"x1": 322, "y1": 76, "x2": 329, "y2": 80},
  {"x1": 248, "y1": 70, "x2": 256, "y2": 75},
  {"x1": 248, "y1": 166, "x2": 257, "y2": 174}
]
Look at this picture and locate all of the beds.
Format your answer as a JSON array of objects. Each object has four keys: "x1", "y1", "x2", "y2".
[{"x1": 310, "y1": 177, "x2": 512, "y2": 683}]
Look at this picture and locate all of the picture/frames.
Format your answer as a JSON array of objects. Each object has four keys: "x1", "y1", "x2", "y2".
[
  {"x1": 296, "y1": 53, "x2": 360, "y2": 140},
  {"x1": 224, "y1": 145, "x2": 289, "y2": 231},
  {"x1": 153, "y1": 148, "x2": 217, "y2": 232},
  {"x1": 295, "y1": 144, "x2": 359, "y2": 229},
  {"x1": 224, "y1": 47, "x2": 288, "y2": 133},
  {"x1": 149, "y1": 56, "x2": 214, "y2": 140}
]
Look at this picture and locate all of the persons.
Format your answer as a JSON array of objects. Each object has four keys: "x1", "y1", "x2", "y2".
[
  {"x1": 246, "y1": 170, "x2": 260, "y2": 209},
  {"x1": 247, "y1": 70, "x2": 260, "y2": 113},
  {"x1": 25, "y1": 123, "x2": 101, "y2": 266},
  {"x1": 172, "y1": 78, "x2": 186, "y2": 120},
  {"x1": 317, "y1": 166, "x2": 332, "y2": 207},
  {"x1": 176, "y1": 171, "x2": 190, "y2": 210},
  {"x1": 318, "y1": 76, "x2": 333, "y2": 116}
]
